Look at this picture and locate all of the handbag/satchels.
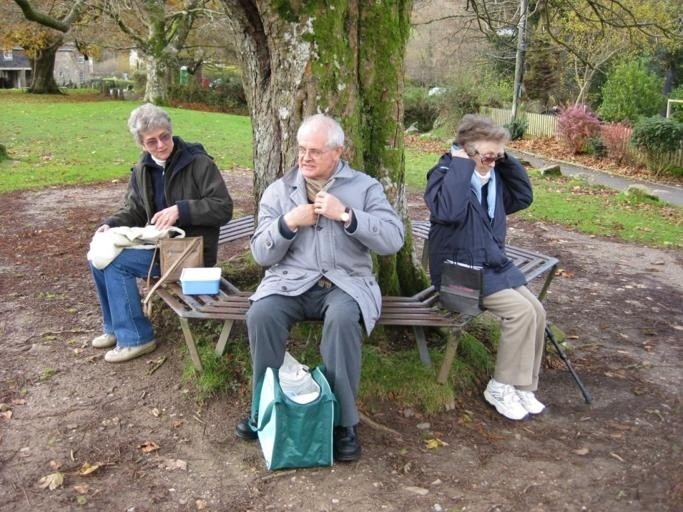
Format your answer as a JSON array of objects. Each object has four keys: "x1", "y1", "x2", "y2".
[
  {"x1": 249, "y1": 363, "x2": 340, "y2": 471},
  {"x1": 157, "y1": 235, "x2": 205, "y2": 284},
  {"x1": 440, "y1": 247, "x2": 483, "y2": 317}
]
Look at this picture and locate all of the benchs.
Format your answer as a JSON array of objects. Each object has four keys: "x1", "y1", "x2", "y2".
[{"x1": 141, "y1": 211, "x2": 558, "y2": 385}]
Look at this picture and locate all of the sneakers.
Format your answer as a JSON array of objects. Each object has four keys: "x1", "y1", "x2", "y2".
[
  {"x1": 483, "y1": 376, "x2": 529, "y2": 421},
  {"x1": 104, "y1": 337, "x2": 157, "y2": 363},
  {"x1": 513, "y1": 387, "x2": 547, "y2": 415},
  {"x1": 92, "y1": 333, "x2": 117, "y2": 349}
]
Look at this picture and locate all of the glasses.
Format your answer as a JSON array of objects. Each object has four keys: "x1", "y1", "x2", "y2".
[
  {"x1": 142, "y1": 130, "x2": 172, "y2": 147},
  {"x1": 469, "y1": 144, "x2": 504, "y2": 166},
  {"x1": 297, "y1": 147, "x2": 330, "y2": 159}
]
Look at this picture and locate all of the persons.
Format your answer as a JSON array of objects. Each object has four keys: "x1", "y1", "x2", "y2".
[
  {"x1": 424, "y1": 115, "x2": 546, "y2": 421},
  {"x1": 235, "y1": 114, "x2": 406, "y2": 461},
  {"x1": 89, "y1": 103, "x2": 233, "y2": 363}
]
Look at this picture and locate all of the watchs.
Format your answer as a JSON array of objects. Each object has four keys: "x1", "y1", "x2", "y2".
[{"x1": 340, "y1": 208, "x2": 349, "y2": 222}]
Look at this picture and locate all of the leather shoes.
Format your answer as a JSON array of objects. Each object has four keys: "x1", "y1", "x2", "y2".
[
  {"x1": 333, "y1": 425, "x2": 362, "y2": 462},
  {"x1": 236, "y1": 414, "x2": 257, "y2": 439}
]
